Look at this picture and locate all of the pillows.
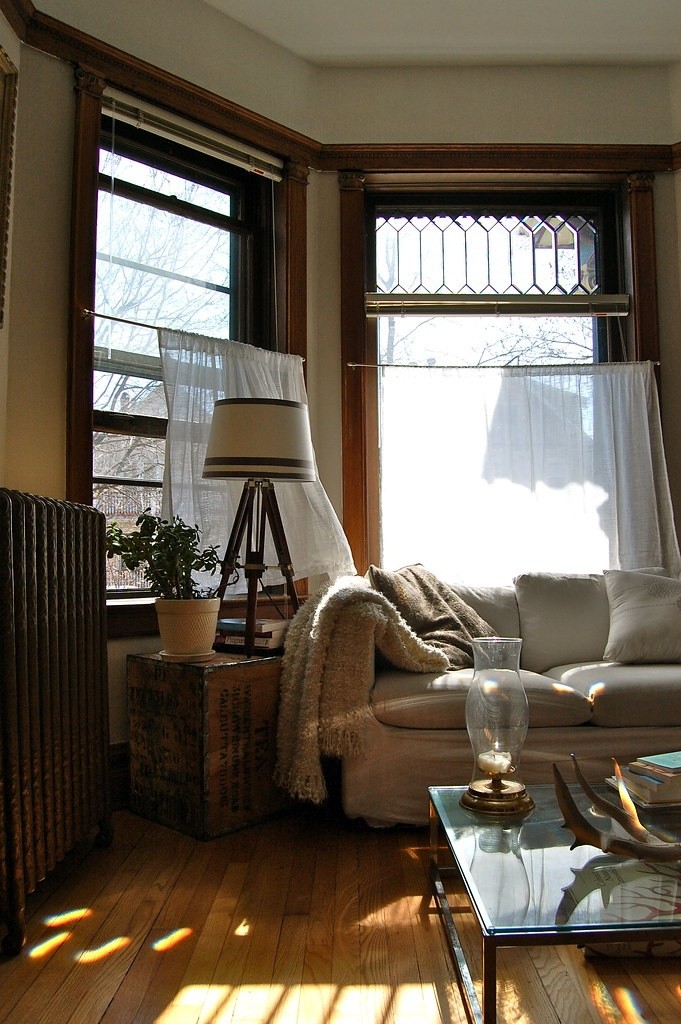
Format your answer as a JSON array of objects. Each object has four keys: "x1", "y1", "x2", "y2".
[
  {"x1": 512, "y1": 566, "x2": 669, "y2": 674},
  {"x1": 602, "y1": 569, "x2": 680, "y2": 668},
  {"x1": 367, "y1": 564, "x2": 507, "y2": 675}
]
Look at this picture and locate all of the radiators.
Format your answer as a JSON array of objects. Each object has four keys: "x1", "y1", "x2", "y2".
[{"x1": 0, "y1": 485, "x2": 116, "y2": 964}]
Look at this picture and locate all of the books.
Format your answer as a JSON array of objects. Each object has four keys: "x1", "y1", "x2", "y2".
[
  {"x1": 603, "y1": 751, "x2": 681, "y2": 809},
  {"x1": 214, "y1": 618, "x2": 287, "y2": 649}
]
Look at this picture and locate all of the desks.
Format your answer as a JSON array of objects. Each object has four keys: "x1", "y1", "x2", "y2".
[
  {"x1": 419, "y1": 776, "x2": 681, "y2": 1024},
  {"x1": 124, "y1": 653, "x2": 282, "y2": 839}
]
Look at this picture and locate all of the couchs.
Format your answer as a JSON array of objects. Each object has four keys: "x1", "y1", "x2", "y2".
[{"x1": 339, "y1": 562, "x2": 681, "y2": 830}]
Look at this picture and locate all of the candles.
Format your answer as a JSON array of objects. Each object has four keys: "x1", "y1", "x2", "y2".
[{"x1": 477, "y1": 747, "x2": 512, "y2": 774}]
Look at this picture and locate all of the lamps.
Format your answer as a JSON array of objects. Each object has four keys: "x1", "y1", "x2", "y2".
[{"x1": 201, "y1": 396, "x2": 320, "y2": 658}]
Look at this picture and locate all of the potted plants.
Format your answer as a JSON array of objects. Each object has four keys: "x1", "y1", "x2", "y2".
[{"x1": 106, "y1": 506, "x2": 243, "y2": 663}]
